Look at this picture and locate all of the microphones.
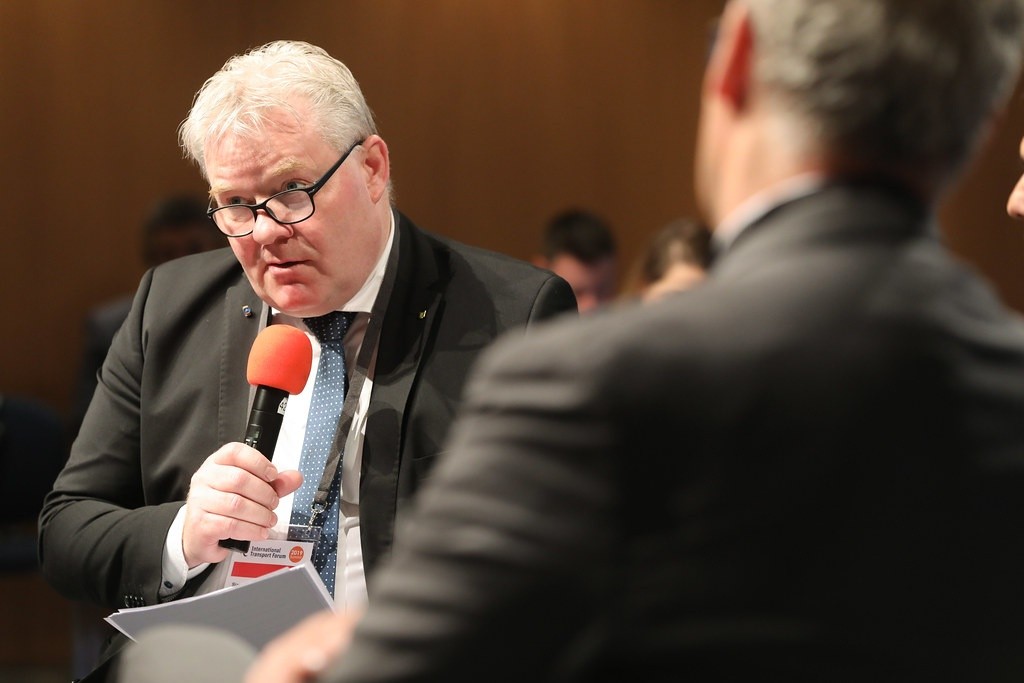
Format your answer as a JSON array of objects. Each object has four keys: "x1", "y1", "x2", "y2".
[{"x1": 216, "y1": 324, "x2": 311, "y2": 556}]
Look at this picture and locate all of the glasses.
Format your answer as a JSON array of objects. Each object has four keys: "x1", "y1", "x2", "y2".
[{"x1": 206, "y1": 140, "x2": 363, "y2": 237}]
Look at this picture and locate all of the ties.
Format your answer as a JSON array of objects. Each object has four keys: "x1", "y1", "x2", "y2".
[{"x1": 286, "y1": 311, "x2": 358, "y2": 602}]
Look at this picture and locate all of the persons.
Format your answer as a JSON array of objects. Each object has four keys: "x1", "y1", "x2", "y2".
[
  {"x1": 631, "y1": 218, "x2": 712, "y2": 299},
  {"x1": 35, "y1": 40, "x2": 579, "y2": 683},
  {"x1": 62, "y1": 198, "x2": 222, "y2": 427},
  {"x1": 323, "y1": 0, "x2": 1024, "y2": 682},
  {"x1": 531, "y1": 209, "x2": 621, "y2": 312}
]
